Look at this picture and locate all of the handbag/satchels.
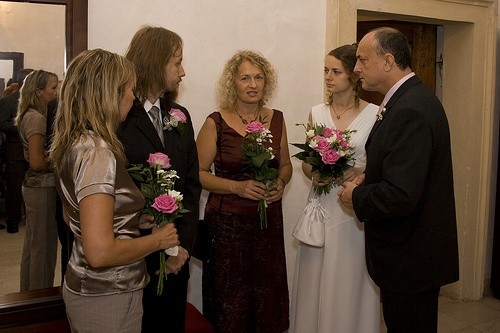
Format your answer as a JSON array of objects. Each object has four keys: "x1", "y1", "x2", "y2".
[{"x1": 190, "y1": 219, "x2": 217, "y2": 262}]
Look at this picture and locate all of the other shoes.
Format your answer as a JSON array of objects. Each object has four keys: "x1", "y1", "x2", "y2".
[{"x1": 7, "y1": 223, "x2": 19, "y2": 233}]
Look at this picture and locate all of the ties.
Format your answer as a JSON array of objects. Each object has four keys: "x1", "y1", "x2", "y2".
[
  {"x1": 148, "y1": 104, "x2": 164, "y2": 142},
  {"x1": 375, "y1": 100, "x2": 384, "y2": 120}
]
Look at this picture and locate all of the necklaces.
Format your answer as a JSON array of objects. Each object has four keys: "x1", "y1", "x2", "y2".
[
  {"x1": 331, "y1": 103, "x2": 354, "y2": 119},
  {"x1": 234, "y1": 107, "x2": 259, "y2": 126}
]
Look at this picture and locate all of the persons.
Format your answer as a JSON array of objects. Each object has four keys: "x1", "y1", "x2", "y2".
[
  {"x1": 290, "y1": 26, "x2": 459, "y2": 333},
  {"x1": 0, "y1": 67, "x2": 60, "y2": 292},
  {"x1": 50, "y1": 25, "x2": 201, "y2": 333},
  {"x1": 196, "y1": 50, "x2": 292, "y2": 333}
]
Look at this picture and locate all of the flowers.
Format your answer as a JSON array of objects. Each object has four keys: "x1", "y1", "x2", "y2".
[
  {"x1": 241, "y1": 114, "x2": 279, "y2": 231},
  {"x1": 164, "y1": 108, "x2": 186, "y2": 131},
  {"x1": 127, "y1": 153, "x2": 190, "y2": 295},
  {"x1": 289, "y1": 120, "x2": 358, "y2": 196}
]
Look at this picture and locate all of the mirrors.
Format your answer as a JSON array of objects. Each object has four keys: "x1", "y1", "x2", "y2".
[{"x1": 0, "y1": 0, "x2": 88, "y2": 326}]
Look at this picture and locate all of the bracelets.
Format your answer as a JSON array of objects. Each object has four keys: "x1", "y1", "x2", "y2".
[{"x1": 279, "y1": 177, "x2": 285, "y2": 187}]
